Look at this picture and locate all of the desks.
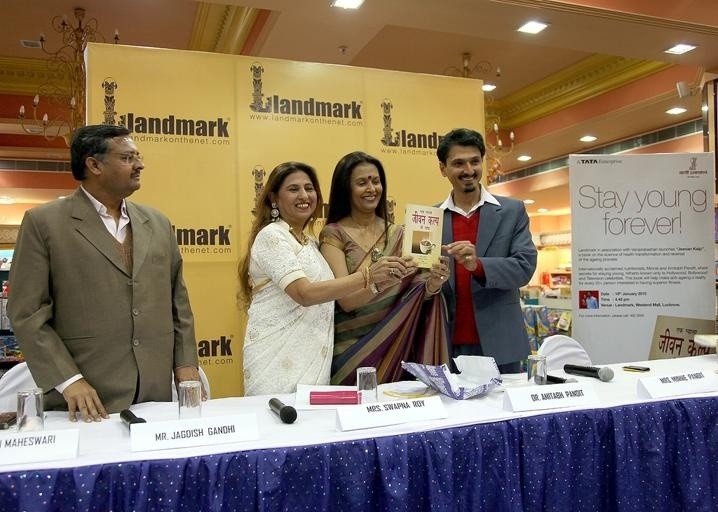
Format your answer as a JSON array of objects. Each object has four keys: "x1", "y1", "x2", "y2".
[{"x1": 0, "y1": 355, "x2": 718, "y2": 512}]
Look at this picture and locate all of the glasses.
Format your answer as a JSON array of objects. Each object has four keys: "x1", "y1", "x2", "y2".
[{"x1": 93, "y1": 150, "x2": 145, "y2": 168}]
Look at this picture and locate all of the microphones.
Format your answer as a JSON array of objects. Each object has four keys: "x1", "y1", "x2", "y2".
[
  {"x1": 547, "y1": 375, "x2": 579, "y2": 382},
  {"x1": 563, "y1": 363, "x2": 615, "y2": 382},
  {"x1": 269, "y1": 398, "x2": 297, "y2": 424},
  {"x1": 118, "y1": 409, "x2": 146, "y2": 432}
]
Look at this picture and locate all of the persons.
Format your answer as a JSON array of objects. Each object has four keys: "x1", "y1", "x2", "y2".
[
  {"x1": 238, "y1": 160, "x2": 409, "y2": 396},
  {"x1": 428, "y1": 127, "x2": 537, "y2": 374},
  {"x1": 319, "y1": 150, "x2": 452, "y2": 386},
  {"x1": 7, "y1": 124, "x2": 209, "y2": 421},
  {"x1": 585, "y1": 291, "x2": 598, "y2": 309}
]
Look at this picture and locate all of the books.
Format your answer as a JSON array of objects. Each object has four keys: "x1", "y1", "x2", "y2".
[{"x1": 402, "y1": 204, "x2": 443, "y2": 269}]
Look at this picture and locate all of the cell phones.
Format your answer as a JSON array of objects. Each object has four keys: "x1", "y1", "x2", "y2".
[{"x1": 623, "y1": 365, "x2": 650, "y2": 372}]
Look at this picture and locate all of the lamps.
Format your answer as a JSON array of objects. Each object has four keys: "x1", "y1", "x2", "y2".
[
  {"x1": 442, "y1": 49, "x2": 515, "y2": 182},
  {"x1": 19, "y1": 8, "x2": 120, "y2": 150}
]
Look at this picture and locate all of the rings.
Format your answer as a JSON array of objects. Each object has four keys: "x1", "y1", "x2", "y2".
[
  {"x1": 440, "y1": 275, "x2": 444, "y2": 281},
  {"x1": 445, "y1": 267, "x2": 448, "y2": 272},
  {"x1": 390, "y1": 268, "x2": 394, "y2": 277}
]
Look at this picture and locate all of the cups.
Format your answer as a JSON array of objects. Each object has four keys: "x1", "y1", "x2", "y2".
[
  {"x1": 357, "y1": 366, "x2": 379, "y2": 404},
  {"x1": 526, "y1": 355, "x2": 548, "y2": 386},
  {"x1": 419, "y1": 240, "x2": 437, "y2": 254},
  {"x1": 17, "y1": 388, "x2": 45, "y2": 433},
  {"x1": 178, "y1": 381, "x2": 202, "y2": 419}
]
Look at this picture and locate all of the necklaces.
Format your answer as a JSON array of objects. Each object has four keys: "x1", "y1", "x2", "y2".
[
  {"x1": 353, "y1": 219, "x2": 384, "y2": 263},
  {"x1": 287, "y1": 227, "x2": 309, "y2": 246}
]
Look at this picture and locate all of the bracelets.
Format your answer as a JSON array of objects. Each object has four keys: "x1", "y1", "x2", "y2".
[
  {"x1": 370, "y1": 284, "x2": 378, "y2": 294},
  {"x1": 424, "y1": 277, "x2": 443, "y2": 296},
  {"x1": 362, "y1": 269, "x2": 375, "y2": 290}
]
